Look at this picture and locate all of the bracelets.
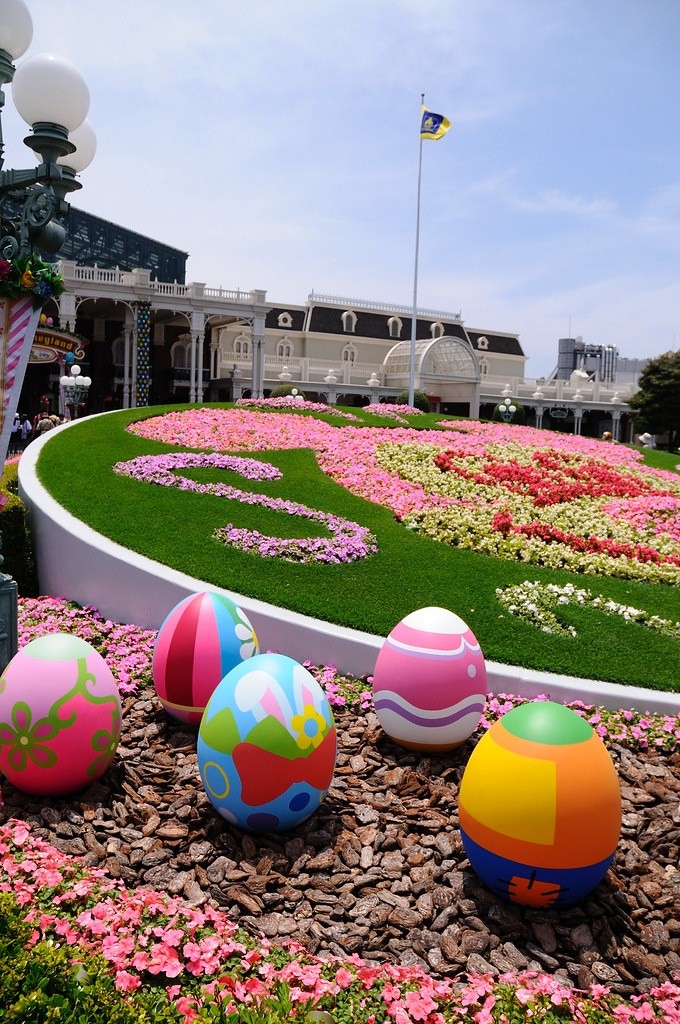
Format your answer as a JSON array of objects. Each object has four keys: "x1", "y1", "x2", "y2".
[{"x1": 31, "y1": 436, "x2": 33, "y2": 437}]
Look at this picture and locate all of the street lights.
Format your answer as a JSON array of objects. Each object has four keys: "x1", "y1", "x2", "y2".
[
  {"x1": 60, "y1": 363, "x2": 92, "y2": 420},
  {"x1": 0, "y1": 1, "x2": 98, "y2": 675}
]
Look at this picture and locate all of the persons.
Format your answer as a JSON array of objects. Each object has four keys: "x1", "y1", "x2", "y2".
[
  {"x1": 20, "y1": 414, "x2": 33, "y2": 452},
  {"x1": 32, "y1": 406, "x2": 68, "y2": 438},
  {"x1": 8, "y1": 412, "x2": 22, "y2": 454},
  {"x1": 638, "y1": 432, "x2": 653, "y2": 449},
  {"x1": 602, "y1": 431, "x2": 613, "y2": 441}
]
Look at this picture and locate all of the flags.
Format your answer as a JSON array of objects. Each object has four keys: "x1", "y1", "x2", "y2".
[{"x1": 420, "y1": 104, "x2": 451, "y2": 141}]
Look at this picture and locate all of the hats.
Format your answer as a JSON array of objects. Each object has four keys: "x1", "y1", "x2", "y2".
[
  {"x1": 14, "y1": 413, "x2": 20, "y2": 418},
  {"x1": 639, "y1": 433, "x2": 651, "y2": 444},
  {"x1": 42, "y1": 412, "x2": 49, "y2": 417},
  {"x1": 22, "y1": 414, "x2": 27, "y2": 418}
]
